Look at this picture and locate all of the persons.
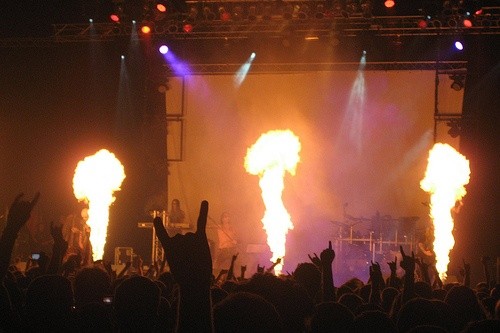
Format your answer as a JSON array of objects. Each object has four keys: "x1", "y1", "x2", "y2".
[
  {"x1": 0, "y1": 191, "x2": 161, "y2": 333},
  {"x1": 169, "y1": 199, "x2": 186, "y2": 223},
  {"x1": 206, "y1": 212, "x2": 254, "y2": 279},
  {"x1": 153, "y1": 200, "x2": 500, "y2": 333},
  {"x1": 71, "y1": 208, "x2": 93, "y2": 266}
]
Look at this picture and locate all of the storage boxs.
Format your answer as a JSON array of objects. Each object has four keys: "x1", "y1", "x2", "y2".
[{"x1": 115, "y1": 246, "x2": 133, "y2": 265}]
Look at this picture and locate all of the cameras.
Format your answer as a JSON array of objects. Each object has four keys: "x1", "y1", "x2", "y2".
[{"x1": 32, "y1": 253, "x2": 40, "y2": 261}]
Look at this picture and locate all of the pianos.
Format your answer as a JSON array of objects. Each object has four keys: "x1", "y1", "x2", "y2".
[{"x1": 137, "y1": 221, "x2": 193, "y2": 231}]
